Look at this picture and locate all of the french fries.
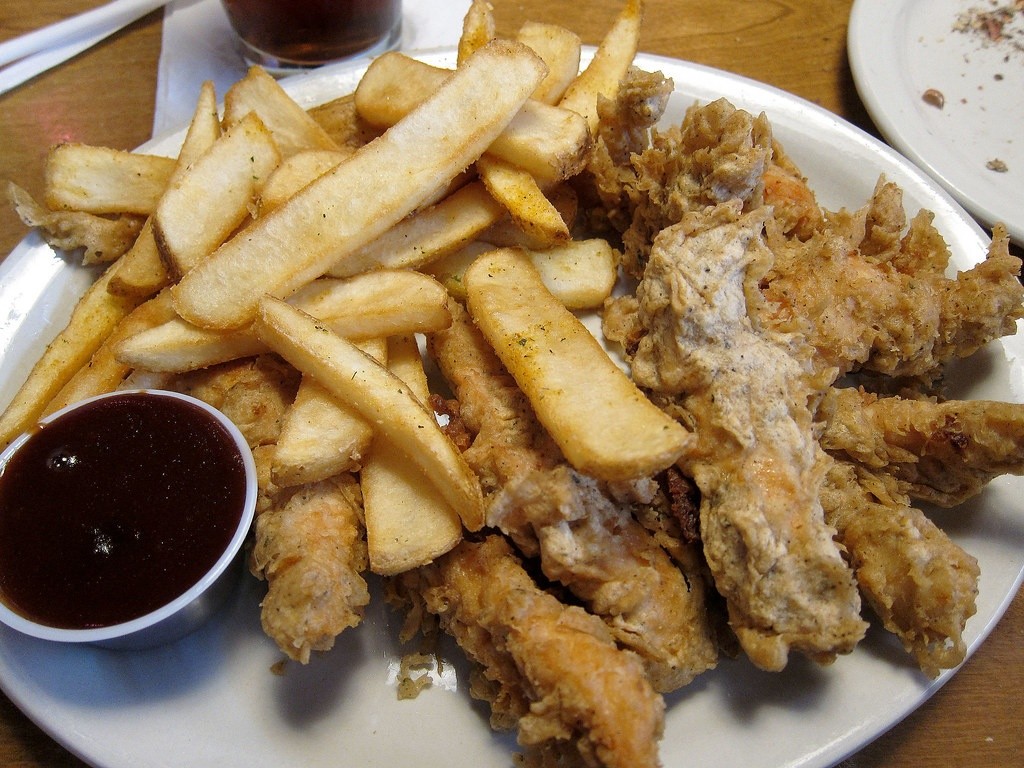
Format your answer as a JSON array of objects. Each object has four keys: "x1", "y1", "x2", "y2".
[{"x1": 1, "y1": 0, "x2": 696, "y2": 572}]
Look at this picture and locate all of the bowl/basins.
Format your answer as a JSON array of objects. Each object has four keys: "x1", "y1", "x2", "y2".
[{"x1": 0, "y1": 390, "x2": 260, "y2": 652}]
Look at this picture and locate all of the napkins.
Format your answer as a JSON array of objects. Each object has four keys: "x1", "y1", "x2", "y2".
[{"x1": 149, "y1": 0, "x2": 476, "y2": 141}]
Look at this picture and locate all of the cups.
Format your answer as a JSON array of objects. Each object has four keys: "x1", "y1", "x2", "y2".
[{"x1": 221, "y1": 0, "x2": 401, "y2": 77}]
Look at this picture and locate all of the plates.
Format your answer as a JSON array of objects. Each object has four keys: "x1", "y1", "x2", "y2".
[
  {"x1": 847, "y1": 0, "x2": 1024, "y2": 242},
  {"x1": 0, "y1": 46, "x2": 1024, "y2": 768}
]
